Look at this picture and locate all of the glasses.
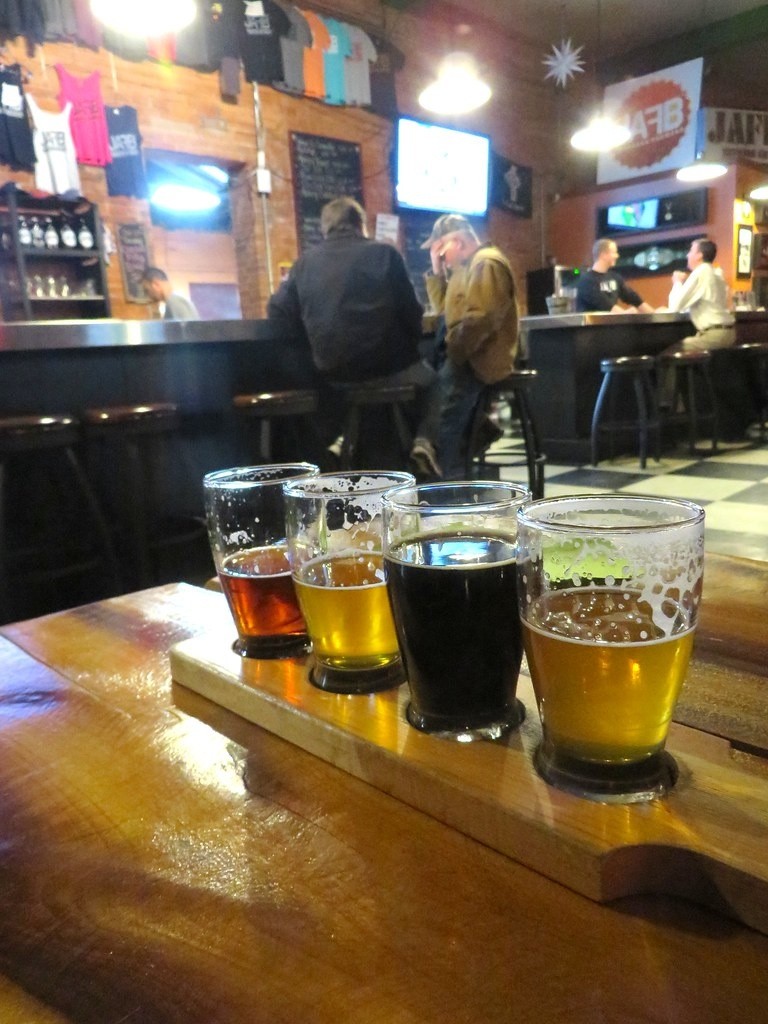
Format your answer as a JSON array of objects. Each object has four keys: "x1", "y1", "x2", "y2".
[{"x1": 439, "y1": 241, "x2": 452, "y2": 259}]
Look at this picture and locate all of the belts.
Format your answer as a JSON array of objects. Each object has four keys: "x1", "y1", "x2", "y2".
[{"x1": 703, "y1": 325, "x2": 733, "y2": 331}]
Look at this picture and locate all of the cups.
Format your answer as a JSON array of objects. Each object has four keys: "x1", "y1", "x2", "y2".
[
  {"x1": 516, "y1": 494, "x2": 707, "y2": 801},
  {"x1": 27, "y1": 272, "x2": 72, "y2": 298},
  {"x1": 381, "y1": 479, "x2": 531, "y2": 741},
  {"x1": 202, "y1": 463, "x2": 321, "y2": 661},
  {"x1": 546, "y1": 297, "x2": 568, "y2": 314},
  {"x1": 282, "y1": 471, "x2": 416, "y2": 694}
]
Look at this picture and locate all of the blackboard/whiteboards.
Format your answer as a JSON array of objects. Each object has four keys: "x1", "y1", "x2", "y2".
[{"x1": 287, "y1": 130, "x2": 365, "y2": 257}]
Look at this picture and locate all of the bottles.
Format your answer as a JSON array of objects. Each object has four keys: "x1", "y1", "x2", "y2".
[{"x1": 18, "y1": 214, "x2": 95, "y2": 251}]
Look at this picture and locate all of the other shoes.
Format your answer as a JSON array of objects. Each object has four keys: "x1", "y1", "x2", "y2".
[
  {"x1": 328, "y1": 436, "x2": 355, "y2": 458},
  {"x1": 411, "y1": 437, "x2": 442, "y2": 480},
  {"x1": 465, "y1": 419, "x2": 504, "y2": 457},
  {"x1": 657, "y1": 397, "x2": 685, "y2": 414}
]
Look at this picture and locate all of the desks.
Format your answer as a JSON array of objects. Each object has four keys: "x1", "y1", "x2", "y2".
[{"x1": 0, "y1": 530, "x2": 768, "y2": 1024}]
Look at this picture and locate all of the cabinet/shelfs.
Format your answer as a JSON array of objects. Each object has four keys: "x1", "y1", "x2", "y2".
[{"x1": 0, "y1": 182, "x2": 111, "y2": 323}]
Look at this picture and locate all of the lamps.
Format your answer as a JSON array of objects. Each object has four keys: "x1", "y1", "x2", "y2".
[
  {"x1": 569, "y1": 0, "x2": 633, "y2": 154},
  {"x1": 418, "y1": 0, "x2": 493, "y2": 118},
  {"x1": 674, "y1": 54, "x2": 729, "y2": 182}
]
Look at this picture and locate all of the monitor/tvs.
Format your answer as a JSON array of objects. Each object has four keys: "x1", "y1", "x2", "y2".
[
  {"x1": 394, "y1": 114, "x2": 491, "y2": 221},
  {"x1": 606, "y1": 198, "x2": 660, "y2": 230}
]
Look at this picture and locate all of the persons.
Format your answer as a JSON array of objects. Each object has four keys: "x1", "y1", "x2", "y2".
[
  {"x1": 137, "y1": 268, "x2": 202, "y2": 320},
  {"x1": 651, "y1": 237, "x2": 736, "y2": 415},
  {"x1": 417, "y1": 213, "x2": 520, "y2": 481},
  {"x1": 574, "y1": 239, "x2": 654, "y2": 312},
  {"x1": 266, "y1": 196, "x2": 446, "y2": 479}
]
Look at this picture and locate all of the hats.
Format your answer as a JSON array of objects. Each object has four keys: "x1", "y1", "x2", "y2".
[{"x1": 420, "y1": 213, "x2": 468, "y2": 250}]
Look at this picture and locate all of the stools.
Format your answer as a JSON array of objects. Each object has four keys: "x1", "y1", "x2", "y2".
[
  {"x1": 587, "y1": 355, "x2": 661, "y2": 470},
  {"x1": 718, "y1": 344, "x2": 768, "y2": 433},
  {"x1": 233, "y1": 391, "x2": 337, "y2": 475},
  {"x1": 86, "y1": 404, "x2": 182, "y2": 591},
  {"x1": 1, "y1": 416, "x2": 111, "y2": 619},
  {"x1": 341, "y1": 385, "x2": 415, "y2": 473},
  {"x1": 662, "y1": 352, "x2": 719, "y2": 458},
  {"x1": 466, "y1": 370, "x2": 546, "y2": 498}
]
